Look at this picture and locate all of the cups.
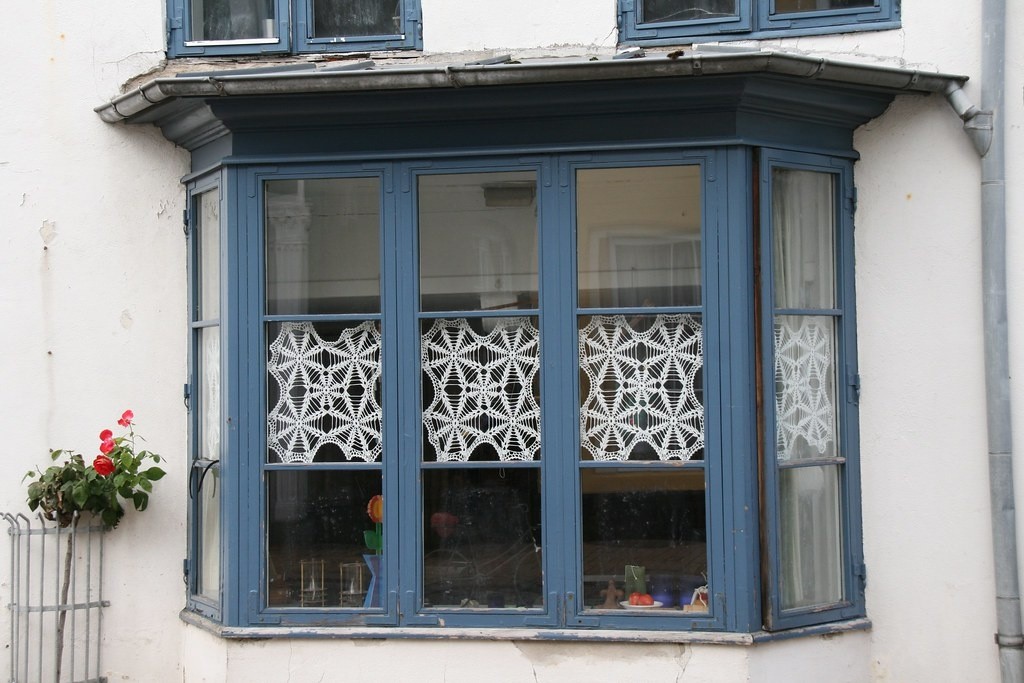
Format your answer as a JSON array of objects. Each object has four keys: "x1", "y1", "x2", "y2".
[
  {"x1": 301, "y1": 560, "x2": 324, "y2": 607},
  {"x1": 339, "y1": 563, "x2": 362, "y2": 608}
]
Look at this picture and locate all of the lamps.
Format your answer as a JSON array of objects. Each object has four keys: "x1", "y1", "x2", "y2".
[{"x1": 481, "y1": 179, "x2": 537, "y2": 207}]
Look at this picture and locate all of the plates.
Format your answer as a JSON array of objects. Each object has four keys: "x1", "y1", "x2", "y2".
[{"x1": 620, "y1": 601, "x2": 663, "y2": 610}]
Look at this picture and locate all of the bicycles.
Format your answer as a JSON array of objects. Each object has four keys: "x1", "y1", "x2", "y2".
[{"x1": 424, "y1": 499, "x2": 545, "y2": 609}]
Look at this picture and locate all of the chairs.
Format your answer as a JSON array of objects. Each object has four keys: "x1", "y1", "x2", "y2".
[
  {"x1": 298, "y1": 559, "x2": 328, "y2": 608},
  {"x1": 338, "y1": 561, "x2": 368, "y2": 608}
]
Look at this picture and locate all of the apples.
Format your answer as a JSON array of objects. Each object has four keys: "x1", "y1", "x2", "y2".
[{"x1": 629, "y1": 592, "x2": 654, "y2": 605}]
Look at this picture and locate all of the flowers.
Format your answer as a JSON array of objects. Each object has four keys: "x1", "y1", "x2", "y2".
[{"x1": 22, "y1": 409, "x2": 169, "y2": 531}]
